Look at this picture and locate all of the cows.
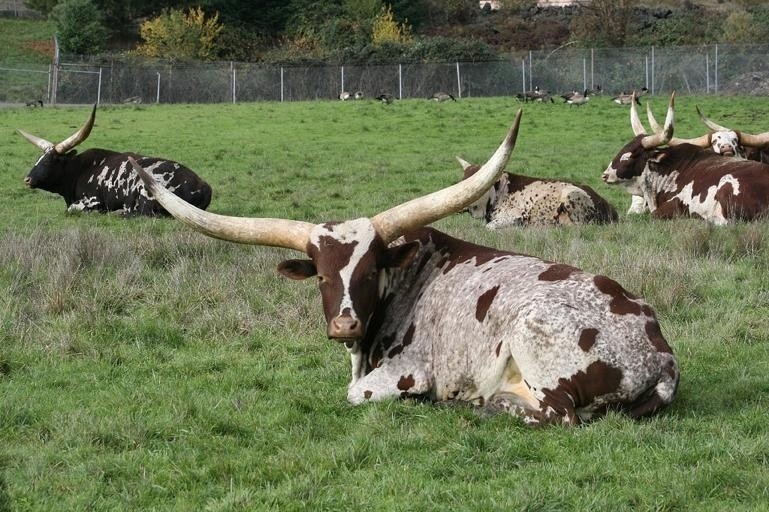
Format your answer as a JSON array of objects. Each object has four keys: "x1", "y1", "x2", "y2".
[
  {"x1": 600, "y1": 90, "x2": 768, "y2": 227},
  {"x1": 15, "y1": 100, "x2": 213, "y2": 221},
  {"x1": 127, "y1": 107, "x2": 682, "y2": 430}
]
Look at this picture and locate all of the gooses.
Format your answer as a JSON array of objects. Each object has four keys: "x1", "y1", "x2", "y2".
[
  {"x1": 26, "y1": 98, "x2": 44, "y2": 108},
  {"x1": 338, "y1": 89, "x2": 366, "y2": 102},
  {"x1": 426, "y1": 90, "x2": 459, "y2": 106},
  {"x1": 120, "y1": 94, "x2": 143, "y2": 105},
  {"x1": 377, "y1": 93, "x2": 400, "y2": 104},
  {"x1": 514, "y1": 84, "x2": 652, "y2": 110}
]
